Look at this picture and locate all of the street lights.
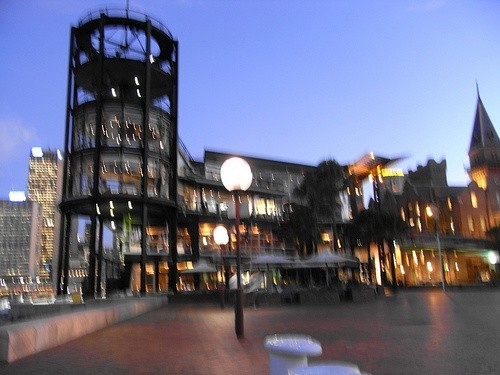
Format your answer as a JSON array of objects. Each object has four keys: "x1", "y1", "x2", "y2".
[
  {"x1": 426, "y1": 203, "x2": 446, "y2": 291},
  {"x1": 220, "y1": 155, "x2": 254, "y2": 337},
  {"x1": 214, "y1": 226, "x2": 227, "y2": 311}
]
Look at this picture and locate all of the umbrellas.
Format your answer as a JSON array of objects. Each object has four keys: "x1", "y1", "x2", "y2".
[
  {"x1": 182, "y1": 260, "x2": 216, "y2": 276},
  {"x1": 245, "y1": 252, "x2": 293, "y2": 269},
  {"x1": 299, "y1": 250, "x2": 356, "y2": 270}
]
